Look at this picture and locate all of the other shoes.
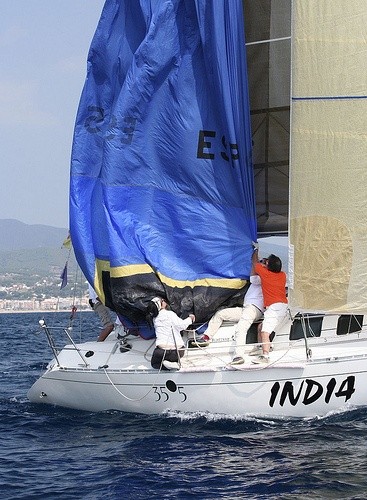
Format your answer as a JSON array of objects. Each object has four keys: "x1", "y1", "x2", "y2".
[
  {"x1": 229, "y1": 357, "x2": 245, "y2": 365},
  {"x1": 191, "y1": 336, "x2": 210, "y2": 346},
  {"x1": 248, "y1": 345, "x2": 264, "y2": 356},
  {"x1": 250, "y1": 355, "x2": 270, "y2": 365}
]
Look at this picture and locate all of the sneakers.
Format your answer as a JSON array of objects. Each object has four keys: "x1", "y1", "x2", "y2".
[{"x1": 162, "y1": 360, "x2": 180, "y2": 370}]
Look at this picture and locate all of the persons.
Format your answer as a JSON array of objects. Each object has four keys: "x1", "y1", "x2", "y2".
[
  {"x1": 252, "y1": 242, "x2": 288, "y2": 364},
  {"x1": 148, "y1": 297, "x2": 195, "y2": 370},
  {"x1": 88, "y1": 285, "x2": 114, "y2": 342},
  {"x1": 192, "y1": 258, "x2": 265, "y2": 365}
]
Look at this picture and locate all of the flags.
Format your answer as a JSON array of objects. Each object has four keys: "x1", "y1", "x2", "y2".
[{"x1": 59, "y1": 234, "x2": 72, "y2": 289}]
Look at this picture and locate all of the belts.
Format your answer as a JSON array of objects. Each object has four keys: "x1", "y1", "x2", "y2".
[{"x1": 252, "y1": 303, "x2": 264, "y2": 316}]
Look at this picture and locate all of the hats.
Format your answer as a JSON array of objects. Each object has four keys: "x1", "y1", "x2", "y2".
[{"x1": 147, "y1": 296, "x2": 162, "y2": 318}]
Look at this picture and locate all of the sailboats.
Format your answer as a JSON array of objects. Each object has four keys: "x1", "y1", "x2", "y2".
[{"x1": 26, "y1": 0, "x2": 367, "y2": 421}]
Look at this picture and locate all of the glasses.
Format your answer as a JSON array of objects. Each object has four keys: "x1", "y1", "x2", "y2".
[
  {"x1": 260, "y1": 260, "x2": 263, "y2": 263},
  {"x1": 162, "y1": 299, "x2": 168, "y2": 303}
]
